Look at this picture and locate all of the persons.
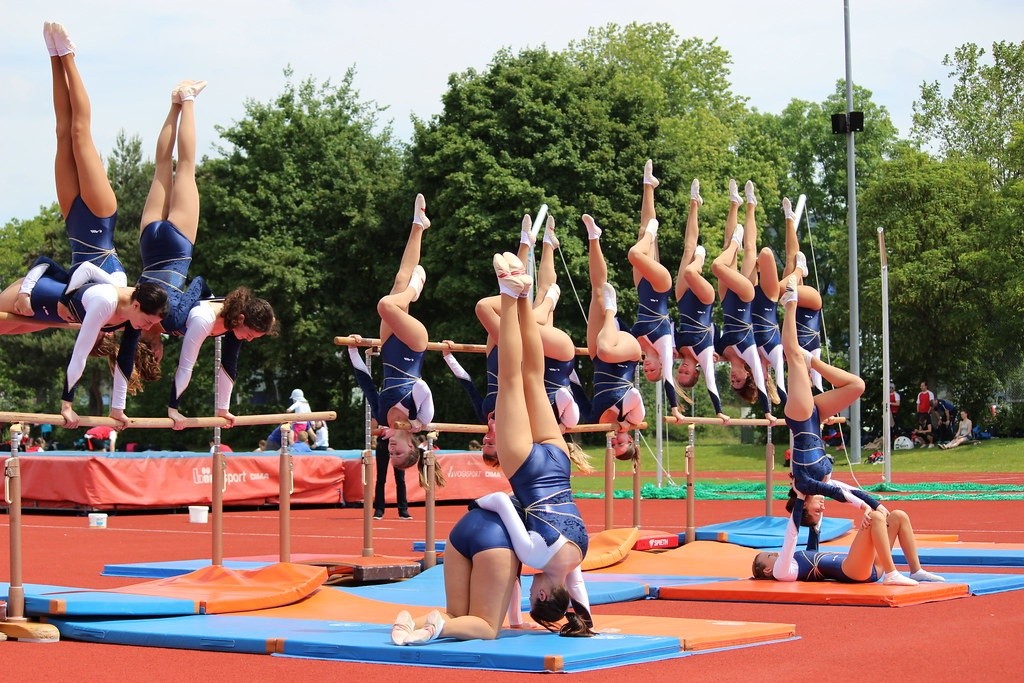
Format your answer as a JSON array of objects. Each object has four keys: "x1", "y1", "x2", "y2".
[
  {"x1": 134, "y1": 79, "x2": 208, "y2": 380},
  {"x1": 937, "y1": 409, "x2": 972, "y2": 449},
  {"x1": 126, "y1": 440, "x2": 139, "y2": 452},
  {"x1": 371, "y1": 435, "x2": 413, "y2": 520},
  {"x1": 581, "y1": 214, "x2": 646, "y2": 475},
  {"x1": 40, "y1": 424, "x2": 52, "y2": 442},
  {"x1": 469, "y1": 213, "x2": 593, "y2": 474},
  {"x1": 749, "y1": 272, "x2": 945, "y2": 587},
  {"x1": 286, "y1": 389, "x2": 312, "y2": 442},
  {"x1": 18, "y1": 424, "x2": 30, "y2": 452},
  {"x1": 347, "y1": 193, "x2": 447, "y2": 488},
  {"x1": 43, "y1": 22, "x2": 145, "y2": 396},
  {"x1": 0, "y1": 276, "x2": 170, "y2": 433},
  {"x1": 628, "y1": 159, "x2": 834, "y2": 426},
  {"x1": 164, "y1": 285, "x2": 275, "y2": 431},
  {"x1": 253, "y1": 419, "x2": 328, "y2": 452},
  {"x1": 48, "y1": 442, "x2": 64, "y2": 451},
  {"x1": 209, "y1": 436, "x2": 233, "y2": 452},
  {"x1": 73, "y1": 427, "x2": 118, "y2": 452},
  {"x1": 392, "y1": 253, "x2": 600, "y2": 646},
  {"x1": 930, "y1": 399, "x2": 958, "y2": 444},
  {"x1": 27, "y1": 437, "x2": 45, "y2": 452},
  {"x1": 935, "y1": 417, "x2": 954, "y2": 444}
]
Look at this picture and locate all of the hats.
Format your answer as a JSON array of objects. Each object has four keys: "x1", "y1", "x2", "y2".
[{"x1": 289, "y1": 389, "x2": 304, "y2": 399}]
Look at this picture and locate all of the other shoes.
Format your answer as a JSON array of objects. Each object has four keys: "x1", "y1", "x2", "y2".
[
  {"x1": 546, "y1": 215, "x2": 559, "y2": 249},
  {"x1": 797, "y1": 343, "x2": 808, "y2": 369},
  {"x1": 408, "y1": 265, "x2": 426, "y2": 302},
  {"x1": 44, "y1": 22, "x2": 75, "y2": 58},
  {"x1": 640, "y1": 159, "x2": 808, "y2": 279},
  {"x1": 392, "y1": 609, "x2": 416, "y2": 646},
  {"x1": 544, "y1": 282, "x2": 560, "y2": 311},
  {"x1": 779, "y1": 274, "x2": 797, "y2": 305},
  {"x1": 171, "y1": 77, "x2": 207, "y2": 102},
  {"x1": 910, "y1": 571, "x2": 944, "y2": 581},
  {"x1": 582, "y1": 214, "x2": 602, "y2": 236},
  {"x1": 522, "y1": 214, "x2": 536, "y2": 246},
  {"x1": 883, "y1": 575, "x2": 918, "y2": 586},
  {"x1": 403, "y1": 609, "x2": 445, "y2": 645},
  {"x1": 601, "y1": 282, "x2": 617, "y2": 314},
  {"x1": 920, "y1": 443, "x2": 949, "y2": 450},
  {"x1": 412, "y1": 194, "x2": 430, "y2": 230},
  {"x1": 492, "y1": 251, "x2": 533, "y2": 294}
]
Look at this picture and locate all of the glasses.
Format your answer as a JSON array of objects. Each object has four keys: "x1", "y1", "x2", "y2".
[{"x1": 890, "y1": 386, "x2": 893, "y2": 389}]
